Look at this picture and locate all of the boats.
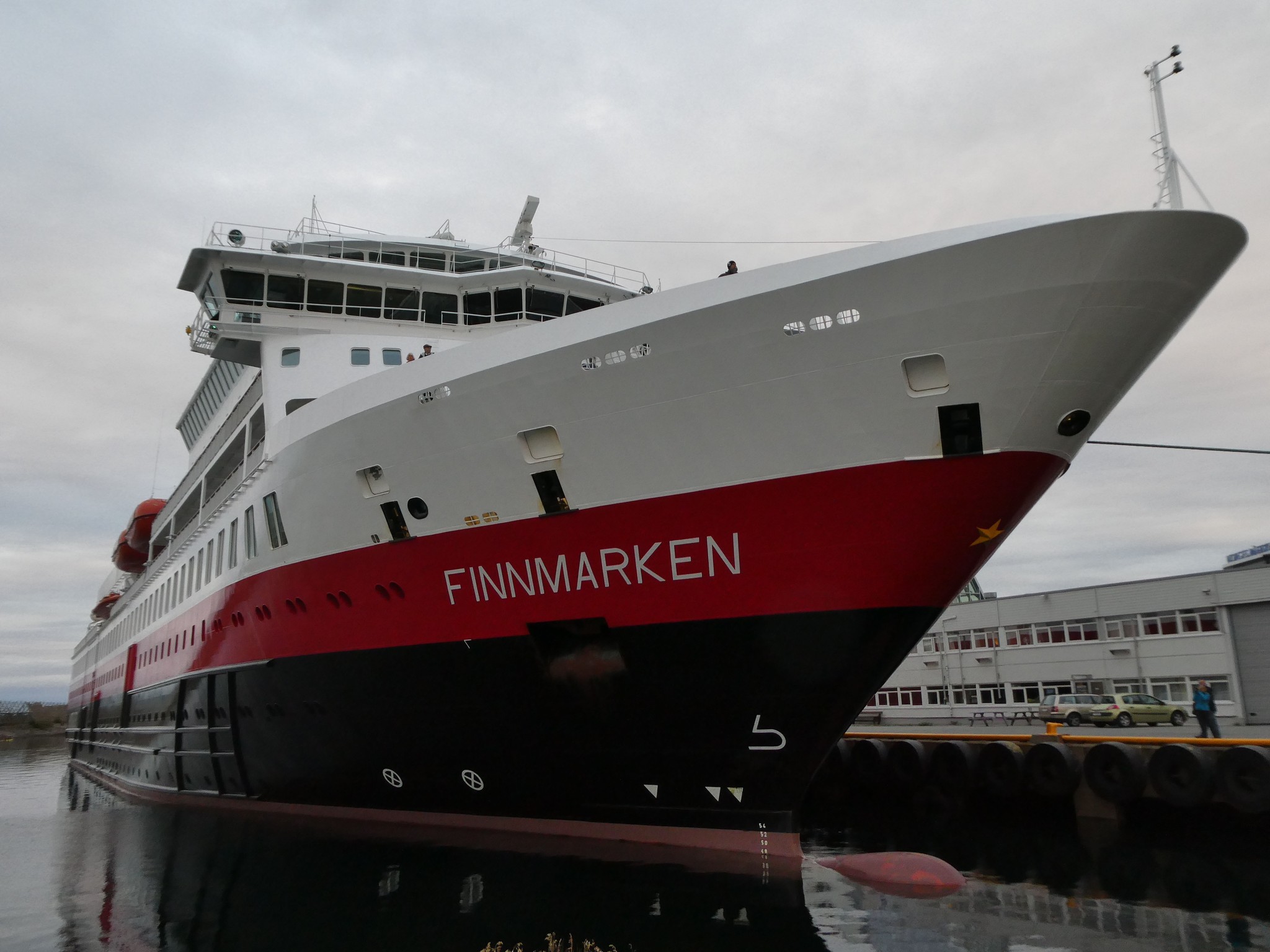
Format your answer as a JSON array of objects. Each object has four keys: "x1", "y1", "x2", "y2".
[
  {"x1": 111, "y1": 529, "x2": 149, "y2": 574},
  {"x1": 92, "y1": 591, "x2": 124, "y2": 619},
  {"x1": 124, "y1": 498, "x2": 174, "y2": 556},
  {"x1": 64, "y1": 44, "x2": 1248, "y2": 952}
]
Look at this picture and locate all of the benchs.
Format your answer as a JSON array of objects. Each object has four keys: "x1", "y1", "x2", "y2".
[
  {"x1": 970, "y1": 717, "x2": 1038, "y2": 720},
  {"x1": 853, "y1": 711, "x2": 883, "y2": 725}
]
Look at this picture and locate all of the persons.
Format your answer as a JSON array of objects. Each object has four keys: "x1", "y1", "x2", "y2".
[
  {"x1": 717, "y1": 260, "x2": 738, "y2": 277},
  {"x1": 1193, "y1": 679, "x2": 1222, "y2": 740},
  {"x1": 418, "y1": 344, "x2": 434, "y2": 359},
  {"x1": 405, "y1": 352, "x2": 415, "y2": 363}
]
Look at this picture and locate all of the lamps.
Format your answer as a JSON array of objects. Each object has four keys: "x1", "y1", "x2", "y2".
[
  {"x1": 227, "y1": 229, "x2": 245, "y2": 247},
  {"x1": 531, "y1": 261, "x2": 545, "y2": 270},
  {"x1": 271, "y1": 241, "x2": 290, "y2": 254},
  {"x1": 639, "y1": 286, "x2": 653, "y2": 294}
]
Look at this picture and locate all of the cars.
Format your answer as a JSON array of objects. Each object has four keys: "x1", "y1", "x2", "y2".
[
  {"x1": 1089, "y1": 693, "x2": 1189, "y2": 728},
  {"x1": 1038, "y1": 694, "x2": 1111, "y2": 727}
]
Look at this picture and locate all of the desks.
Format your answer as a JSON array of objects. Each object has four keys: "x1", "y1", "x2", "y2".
[
  {"x1": 1011, "y1": 710, "x2": 1039, "y2": 726},
  {"x1": 970, "y1": 711, "x2": 1009, "y2": 727}
]
